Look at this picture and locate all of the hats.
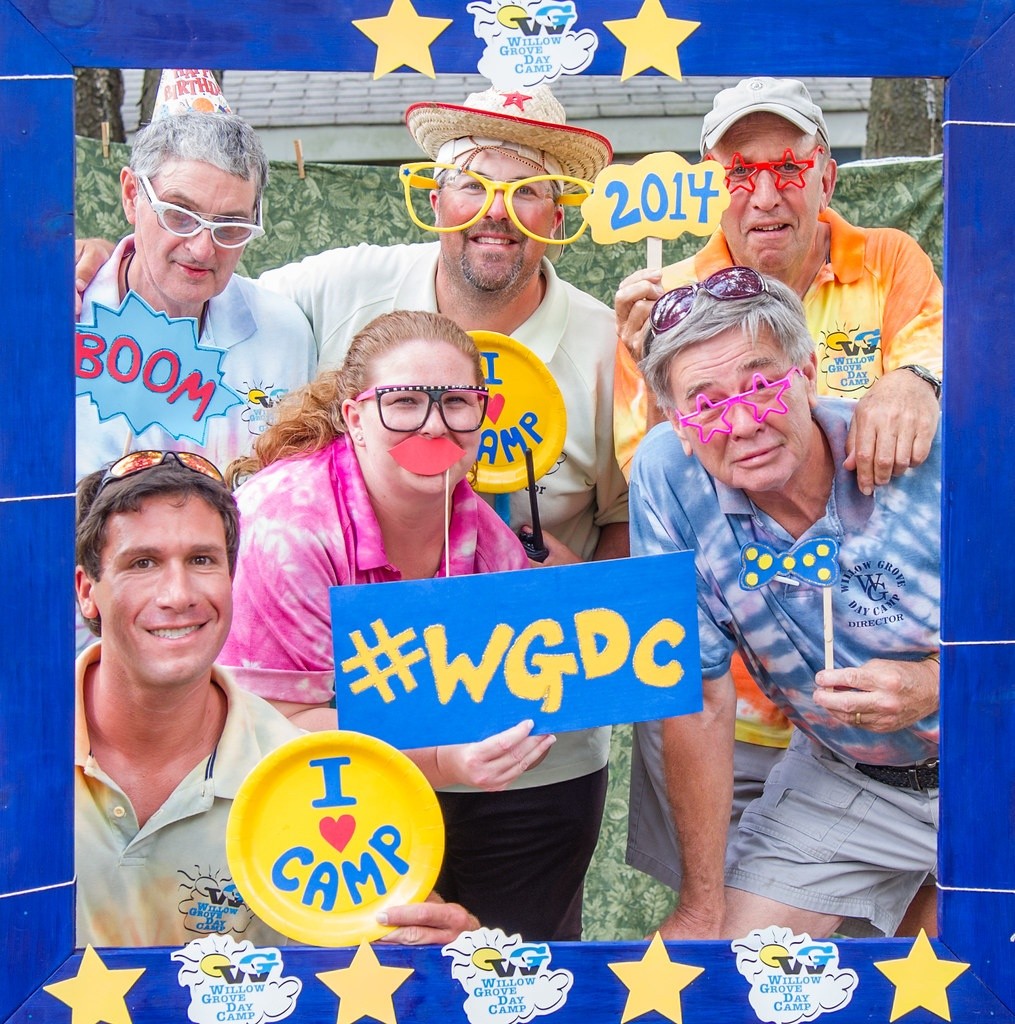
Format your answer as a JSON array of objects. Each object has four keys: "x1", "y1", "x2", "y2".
[
  {"x1": 699, "y1": 77, "x2": 831, "y2": 156},
  {"x1": 405, "y1": 82, "x2": 614, "y2": 194}
]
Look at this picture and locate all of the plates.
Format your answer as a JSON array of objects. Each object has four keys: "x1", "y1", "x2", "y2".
[
  {"x1": 217, "y1": 723, "x2": 448, "y2": 952},
  {"x1": 451, "y1": 330, "x2": 566, "y2": 493}
]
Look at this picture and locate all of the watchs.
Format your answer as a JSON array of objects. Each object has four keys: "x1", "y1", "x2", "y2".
[{"x1": 892, "y1": 363, "x2": 941, "y2": 398}]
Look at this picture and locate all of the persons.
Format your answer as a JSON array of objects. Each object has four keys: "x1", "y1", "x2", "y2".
[
  {"x1": 72, "y1": 106, "x2": 322, "y2": 656},
  {"x1": 625, "y1": 263, "x2": 942, "y2": 947},
  {"x1": 73, "y1": 78, "x2": 635, "y2": 946},
  {"x1": 63, "y1": 450, "x2": 483, "y2": 950},
  {"x1": 614, "y1": 76, "x2": 944, "y2": 942},
  {"x1": 211, "y1": 310, "x2": 560, "y2": 794}
]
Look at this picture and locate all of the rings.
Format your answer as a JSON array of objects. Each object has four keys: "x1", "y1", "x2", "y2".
[{"x1": 855, "y1": 711, "x2": 862, "y2": 726}]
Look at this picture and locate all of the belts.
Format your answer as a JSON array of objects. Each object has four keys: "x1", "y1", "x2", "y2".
[{"x1": 830, "y1": 753, "x2": 939, "y2": 791}]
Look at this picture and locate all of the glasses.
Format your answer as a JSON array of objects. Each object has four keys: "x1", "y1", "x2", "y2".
[
  {"x1": 706, "y1": 145, "x2": 825, "y2": 194},
  {"x1": 399, "y1": 161, "x2": 597, "y2": 245},
  {"x1": 136, "y1": 173, "x2": 265, "y2": 249},
  {"x1": 670, "y1": 367, "x2": 805, "y2": 443},
  {"x1": 644, "y1": 266, "x2": 785, "y2": 356},
  {"x1": 354, "y1": 384, "x2": 489, "y2": 432},
  {"x1": 93, "y1": 449, "x2": 229, "y2": 488}
]
